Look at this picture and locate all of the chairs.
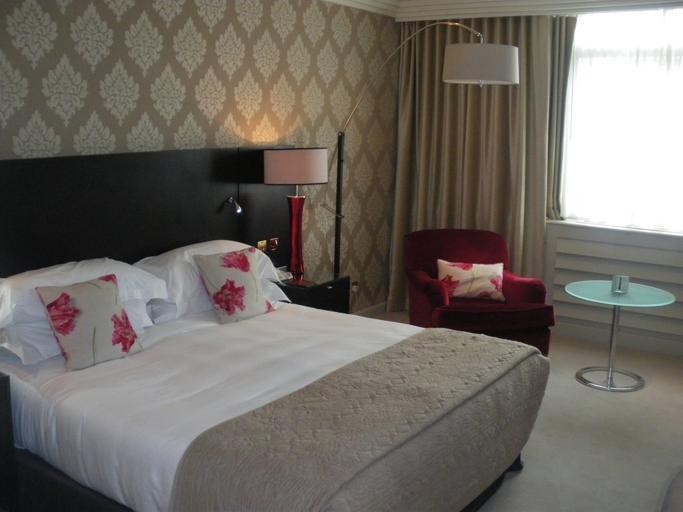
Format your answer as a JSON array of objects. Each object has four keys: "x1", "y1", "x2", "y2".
[{"x1": 403, "y1": 228, "x2": 554, "y2": 355}]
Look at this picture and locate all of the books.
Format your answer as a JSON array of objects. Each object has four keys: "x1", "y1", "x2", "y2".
[{"x1": 282, "y1": 277, "x2": 317, "y2": 288}]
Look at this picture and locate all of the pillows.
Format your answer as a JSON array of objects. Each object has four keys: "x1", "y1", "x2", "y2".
[
  {"x1": 436, "y1": 258, "x2": 506, "y2": 303},
  {"x1": 0, "y1": 238, "x2": 294, "y2": 370}
]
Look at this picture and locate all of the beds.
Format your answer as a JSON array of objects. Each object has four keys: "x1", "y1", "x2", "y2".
[{"x1": 0, "y1": 299, "x2": 550, "y2": 512}]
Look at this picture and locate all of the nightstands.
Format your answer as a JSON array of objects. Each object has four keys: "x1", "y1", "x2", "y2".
[{"x1": 276, "y1": 270, "x2": 350, "y2": 313}]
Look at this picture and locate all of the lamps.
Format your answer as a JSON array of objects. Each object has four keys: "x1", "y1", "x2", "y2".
[
  {"x1": 321, "y1": 22, "x2": 518, "y2": 273},
  {"x1": 262, "y1": 147, "x2": 328, "y2": 275}
]
